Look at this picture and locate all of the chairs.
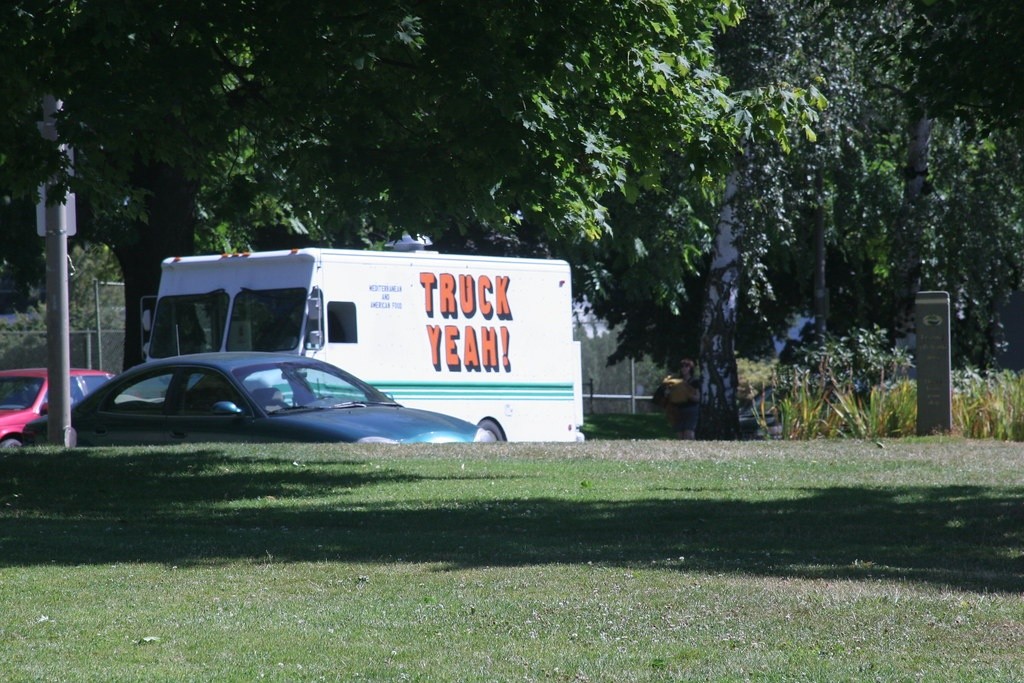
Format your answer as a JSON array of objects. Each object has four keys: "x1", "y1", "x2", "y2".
[
  {"x1": 255, "y1": 302, "x2": 297, "y2": 351},
  {"x1": 3, "y1": 390, "x2": 25, "y2": 405},
  {"x1": 328, "y1": 311, "x2": 345, "y2": 342},
  {"x1": 251, "y1": 387, "x2": 285, "y2": 413},
  {"x1": 185, "y1": 387, "x2": 233, "y2": 412}
]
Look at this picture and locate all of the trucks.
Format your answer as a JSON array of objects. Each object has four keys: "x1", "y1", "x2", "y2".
[{"x1": 138, "y1": 233, "x2": 587, "y2": 442}]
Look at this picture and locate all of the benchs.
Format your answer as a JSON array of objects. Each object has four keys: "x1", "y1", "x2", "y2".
[{"x1": 118, "y1": 401, "x2": 166, "y2": 412}]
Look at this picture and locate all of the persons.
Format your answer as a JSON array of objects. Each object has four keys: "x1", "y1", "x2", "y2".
[
  {"x1": 253, "y1": 288, "x2": 345, "y2": 352},
  {"x1": 662, "y1": 358, "x2": 702, "y2": 441}
]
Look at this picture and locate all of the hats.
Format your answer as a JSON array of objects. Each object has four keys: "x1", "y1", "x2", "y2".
[{"x1": 680, "y1": 358, "x2": 694, "y2": 367}]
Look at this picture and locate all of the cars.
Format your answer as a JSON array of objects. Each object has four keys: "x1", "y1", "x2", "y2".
[
  {"x1": 0, "y1": 367, "x2": 143, "y2": 448},
  {"x1": 21, "y1": 349, "x2": 490, "y2": 444}
]
